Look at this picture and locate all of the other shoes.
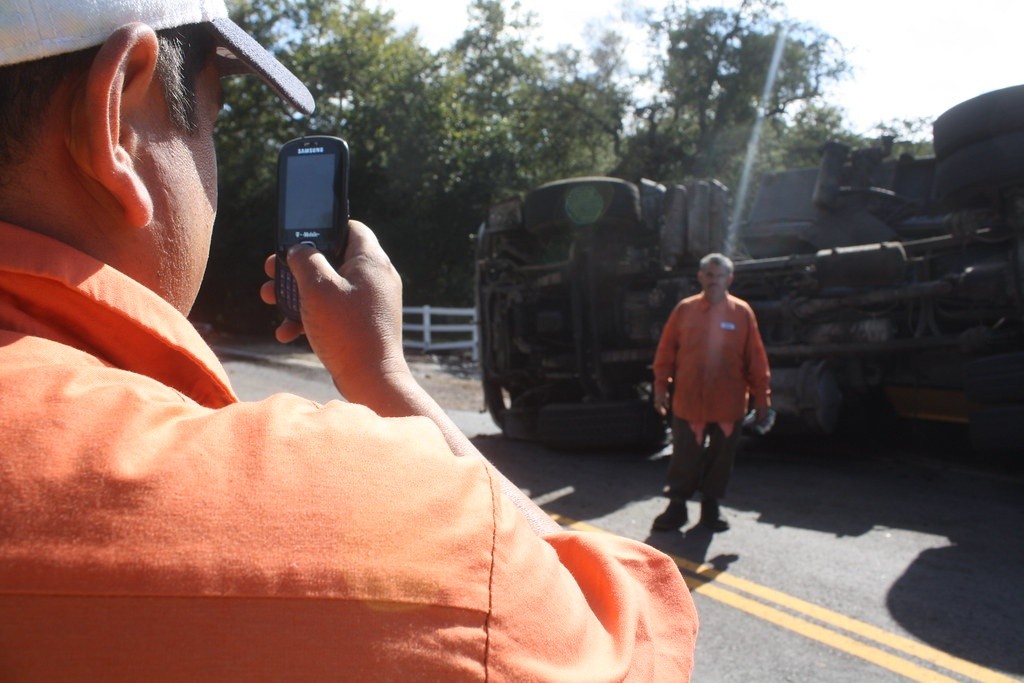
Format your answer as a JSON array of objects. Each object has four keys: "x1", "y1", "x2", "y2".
[
  {"x1": 700, "y1": 516, "x2": 728, "y2": 533},
  {"x1": 653, "y1": 506, "x2": 688, "y2": 531}
]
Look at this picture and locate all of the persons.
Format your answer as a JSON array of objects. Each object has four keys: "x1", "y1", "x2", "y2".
[
  {"x1": 1, "y1": 0, "x2": 699, "y2": 683},
  {"x1": 653, "y1": 254, "x2": 773, "y2": 534}
]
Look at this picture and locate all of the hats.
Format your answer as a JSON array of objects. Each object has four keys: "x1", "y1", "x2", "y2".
[{"x1": 0, "y1": 0, "x2": 317, "y2": 117}]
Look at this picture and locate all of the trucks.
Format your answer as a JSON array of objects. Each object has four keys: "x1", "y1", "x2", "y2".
[{"x1": 474, "y1": 80, "x2": 1023, "y2": 453}]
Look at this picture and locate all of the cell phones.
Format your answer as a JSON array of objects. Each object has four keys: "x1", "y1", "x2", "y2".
[{"x1": 274, "y1": 135, "x2": 349, "y2": 323}]
